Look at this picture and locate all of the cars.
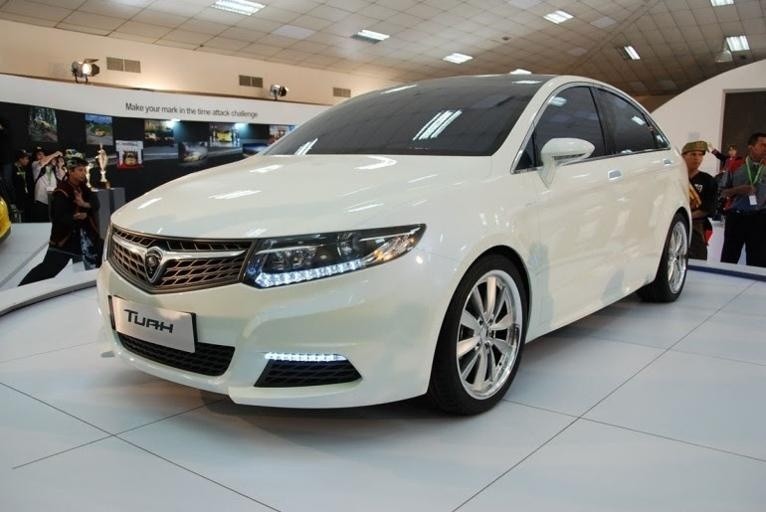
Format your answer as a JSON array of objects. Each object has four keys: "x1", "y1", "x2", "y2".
[
  {"x1": 95, "y1": 129, "x2": 105, "y2": 137},
  {"x1": 218, "y1": 131, "x2": 235, "y2": 142},
  {"x1": 33, "y1": 116, "x2": 50, "y2": 130},
  {"x1": 96, "y1": 73, "x2": 694, "y2": 416},
  {"x1": 183, "y1": 151, "x2": 202, "y2": 162},
  {"x1": 64, "y1": 149, "x2": 84, "y2": 162}
]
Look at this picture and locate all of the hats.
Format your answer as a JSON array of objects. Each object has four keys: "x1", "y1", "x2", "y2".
[
  {"x1": 64, "y1": 156, "x2": 89, "y2": 170},
  {"x1": 679, "y1": 140, "x2": 709, "y2": 156}
]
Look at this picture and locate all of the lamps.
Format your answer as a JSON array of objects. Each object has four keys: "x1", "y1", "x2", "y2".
[
  {"x1": 271, "y1": 85, "x2": 286, "y2": 101},
  {"x1": 71, "y1": 59, "x2": 92, "y2": 85}
]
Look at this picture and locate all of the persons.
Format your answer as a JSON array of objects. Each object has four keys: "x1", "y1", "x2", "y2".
[
  {"x1": 718, "y1": 133, "x2": 766, "y2": 267},
  {"x1": 681, "y1": 141, "x2": 718, "y2": 260},
  {"x1": 710, "y1": 141, "x2": 744, "y2": 222},
  {"x1": 704, "y1": 145, "x2": 729, "y2": 172},
  {"x1": 0, "y1": 143, "x2": 109, "y2": 287}
]
